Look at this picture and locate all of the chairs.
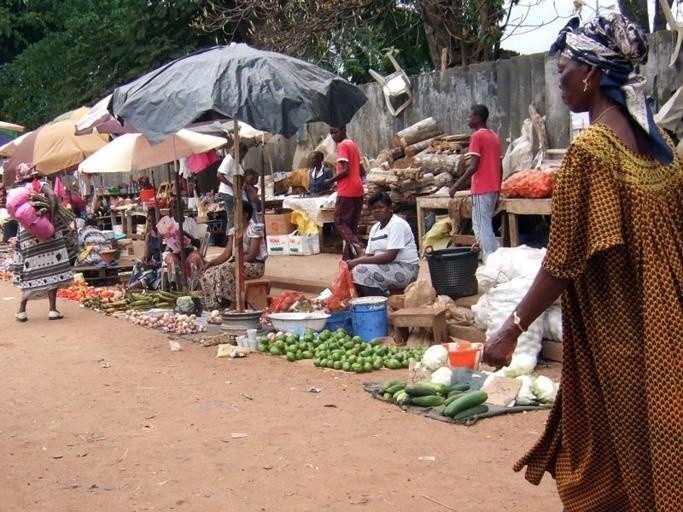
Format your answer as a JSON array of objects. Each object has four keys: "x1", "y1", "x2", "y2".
[
  {"x1": 659, "y1": 0, "x2": 683, "y2": 67},
  {"x1": 368, "y1": 54, "x2": 414, "y2": 117},
  {"x1": 160, "y1": 222, "x2": 211, "y2": 291}
]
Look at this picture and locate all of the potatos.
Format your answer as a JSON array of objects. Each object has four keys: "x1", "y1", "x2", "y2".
[{"x1": 207, "y1": 309, "x2": 222, "y2": 325}]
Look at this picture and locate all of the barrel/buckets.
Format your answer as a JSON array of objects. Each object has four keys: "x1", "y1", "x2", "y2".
[
  {"x1": 139, "y1": 189, "x2": 154, "y2": 203},
  {"x1": 348, "y1": 295, "x2": 389, "y2": 342}
]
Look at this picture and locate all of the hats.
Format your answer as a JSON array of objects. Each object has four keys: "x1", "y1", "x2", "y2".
[{"x1": 13, "y1": 162, "x2": 38, "y2": 183}]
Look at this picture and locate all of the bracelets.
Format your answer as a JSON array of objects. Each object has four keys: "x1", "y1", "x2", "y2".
[{"x1": 512, "y1": 310, "x2": 525, "y2": 333}]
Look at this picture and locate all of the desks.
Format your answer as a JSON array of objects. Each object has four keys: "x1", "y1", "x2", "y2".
[
  {"x1": 415, "y1": 195, "x2": 553, "y2": 259},
  {"x1": 319, "y1": 208, "x2": 337, "y2": 252},
  {"x1": 96, "y1": 207, "x2": 172, "y2": 240}
]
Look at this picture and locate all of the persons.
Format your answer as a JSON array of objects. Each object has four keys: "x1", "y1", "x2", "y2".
[
  {"x1": 447, "y1": 104, "x2": 501, "y2": 262},
  {"x1": 478, "y1": 12, "x2": 683, "y2": 509}
]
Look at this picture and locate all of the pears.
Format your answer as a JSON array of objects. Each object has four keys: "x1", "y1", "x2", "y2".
[{"x1": 258, "y1": 327, "x2": 425, "y2": 374}]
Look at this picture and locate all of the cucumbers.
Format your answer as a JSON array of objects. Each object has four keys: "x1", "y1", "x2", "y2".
[{"x1": 380, "y1": 378, "x2": 488, "y2": 420}]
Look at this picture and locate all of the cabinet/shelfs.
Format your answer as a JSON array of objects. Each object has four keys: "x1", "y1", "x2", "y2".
[{"x1": 72, "y1": 255, "x2": 134, "y2": 283}]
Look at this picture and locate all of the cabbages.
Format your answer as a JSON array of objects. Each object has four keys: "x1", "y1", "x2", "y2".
[{"x1": 422, "y1": 344, "x2": 559, "y2": 405}]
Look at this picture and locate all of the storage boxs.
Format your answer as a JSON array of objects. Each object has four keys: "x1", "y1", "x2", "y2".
[{"x1": 263, "y1": 207, "x2": 321, "y2": 256}]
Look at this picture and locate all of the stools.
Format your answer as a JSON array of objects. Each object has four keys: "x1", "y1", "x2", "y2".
[
  {"x1": 242, "y1": 278, "x2": 273, "y2": 311},
  {"x1": 389, "y1": 305, "x2": 449, "y2": 345}
]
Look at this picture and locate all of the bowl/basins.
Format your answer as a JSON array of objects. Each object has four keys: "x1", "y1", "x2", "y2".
[
  {"x1": 440, "y1": 342, "x2": 484, "y2": 367},
  {"x1": 267, "y1": 312, "x2": 332, "y2": 331},
  {"x1": 146, "y1": 201, "x2": 166, "y2": 208}
]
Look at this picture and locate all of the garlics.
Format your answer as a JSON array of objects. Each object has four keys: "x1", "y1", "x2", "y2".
[{"x1": 123, "y1": 308, "x2": 207, "y2": 335}]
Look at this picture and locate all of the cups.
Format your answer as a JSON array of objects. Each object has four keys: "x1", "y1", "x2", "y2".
[{"x1": 235, "y1": 328, "x2": 260, "y2": 351}]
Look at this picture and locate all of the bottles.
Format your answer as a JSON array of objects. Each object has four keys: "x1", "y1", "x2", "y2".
[{"x1": 128, "y1": 180, "x2": 141, "y2": 194}]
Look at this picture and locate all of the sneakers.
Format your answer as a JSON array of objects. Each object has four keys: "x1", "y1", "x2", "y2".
[
  {"x1": 48, "y1": 309, "x2": 64, "y2": 319},
  {"x1": 15, "y1": 311, "x2": 27, "y2": 321}
]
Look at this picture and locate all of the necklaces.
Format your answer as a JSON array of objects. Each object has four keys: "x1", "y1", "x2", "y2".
[{"x1": 590, "y1": 105, "x2": 623, "y2": 126}]
